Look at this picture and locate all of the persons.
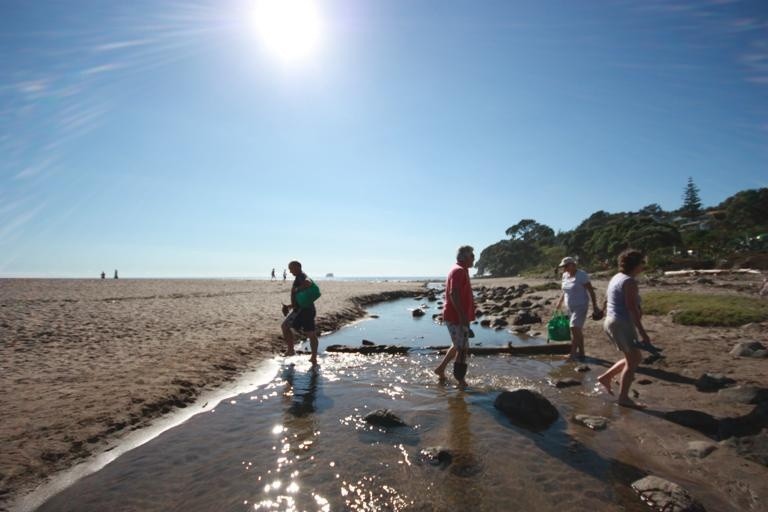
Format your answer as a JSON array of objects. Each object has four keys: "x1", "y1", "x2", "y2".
[
  {"x1": 598, "y1": 249, "x2": 650, "y2": 409},
  {"x1": 283, "y1": 271, "x2": 287, "y2": 280},
  {"x1": 271, "y1": 269, "x2": 277, "y2": 281},
  {"x1": 434, "y1": 246, "x2": 475, "y2": 386},
  {"x1": 281, "y1": 261, "x2": 319, "y2": 363},
  {"x1": 553, "y1": 258, "x2": 599, "y2": 359}
]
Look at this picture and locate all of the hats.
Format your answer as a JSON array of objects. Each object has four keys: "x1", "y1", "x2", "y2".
[{"x1": 558, "y1": 256, "x2": 574, "y2": 267}]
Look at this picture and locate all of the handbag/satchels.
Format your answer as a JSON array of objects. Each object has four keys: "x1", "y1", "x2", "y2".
[
  {"x1": 549, "y1": 309, "x2": 571, "y2": 340},
  {"x1": 295, "y1": 277, "x2": 321, "y2": 309}
]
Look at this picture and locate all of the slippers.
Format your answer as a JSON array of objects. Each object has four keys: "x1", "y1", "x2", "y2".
[{"x1": 635, "y1": 341, "x2": 661, "y2": 356}]
